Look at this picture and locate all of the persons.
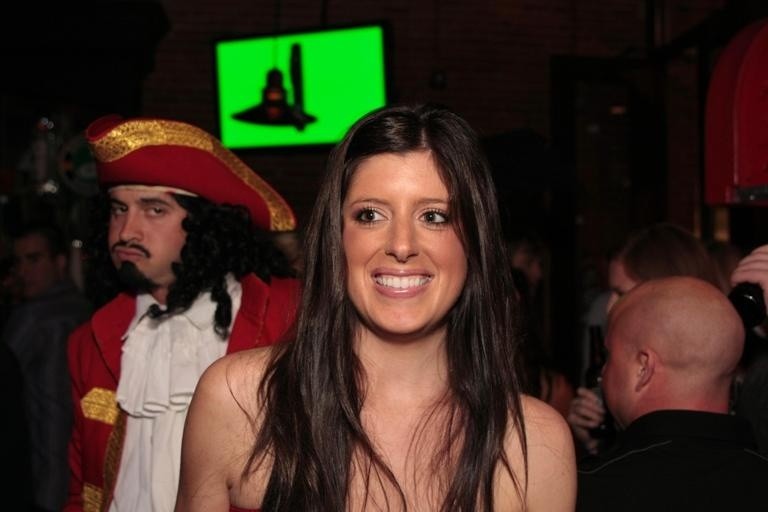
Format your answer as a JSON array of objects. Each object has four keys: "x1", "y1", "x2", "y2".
[{"x1": 0, "y1": 101, "x2": 768, "y2": 512}]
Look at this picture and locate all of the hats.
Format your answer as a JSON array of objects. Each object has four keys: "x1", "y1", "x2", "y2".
[{"x1": 83, "y1": 114, "x2": 298, "y2": 234}]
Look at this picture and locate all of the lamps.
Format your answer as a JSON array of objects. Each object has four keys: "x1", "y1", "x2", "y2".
[{"x1": 230, "y1": 35, "x2": 317, "y2": 126}]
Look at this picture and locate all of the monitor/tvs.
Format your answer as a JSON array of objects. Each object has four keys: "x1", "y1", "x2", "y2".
[{"x1": 209, "y1": 19, "x2": 396, "y2": 158}]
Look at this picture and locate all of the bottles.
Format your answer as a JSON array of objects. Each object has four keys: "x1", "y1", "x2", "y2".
[
  {"x1": 727, "y1": 280, "x2": 766, "y2": 329},
  {"x1": 68, "y1": 237, "x2": 97, "y2": 298}
]
